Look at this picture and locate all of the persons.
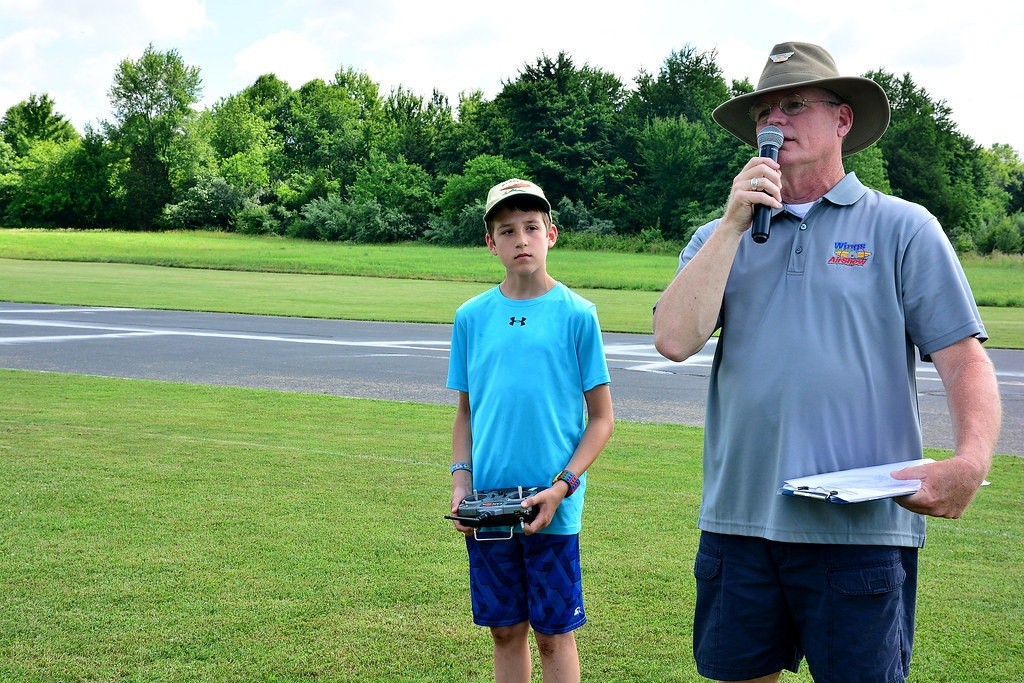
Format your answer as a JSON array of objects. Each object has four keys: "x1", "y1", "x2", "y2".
[
  {"x1": 651, "y1": 41, "x2": 1001, "y2": 683},
  {"x1": 446, "y1": 178, "x2": 614, "y2": 683}
]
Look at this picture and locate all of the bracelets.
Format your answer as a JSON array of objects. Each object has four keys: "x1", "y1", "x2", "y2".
[
  {"x1": 451, "y1": 462, "x2": 472, "y2": 476},
  {"x1": 552, "y1": 470, "x2": 581, "y2": 498}
]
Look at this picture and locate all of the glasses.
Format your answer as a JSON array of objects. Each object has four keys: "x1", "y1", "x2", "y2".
[{"x1": 746, "y1": 93, "x2": 840, "y2": 123}]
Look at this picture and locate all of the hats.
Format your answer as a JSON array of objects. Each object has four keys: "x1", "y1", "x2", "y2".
[
  {"x1": 711, "y1": 42, "x2": 891, "y2": 158},
  {"x1": 483, "y1": 178, "x2": 552, "y2": 233}
]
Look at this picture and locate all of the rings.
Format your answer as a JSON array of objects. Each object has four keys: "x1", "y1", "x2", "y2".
[{"x1": 750, "y1": 177, "x2": 758, "y2": 191}]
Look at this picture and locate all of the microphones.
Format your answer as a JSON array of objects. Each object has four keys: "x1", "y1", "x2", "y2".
[{"x1": 751, "y1": 124, "x2": 785, "y2": 244}]
